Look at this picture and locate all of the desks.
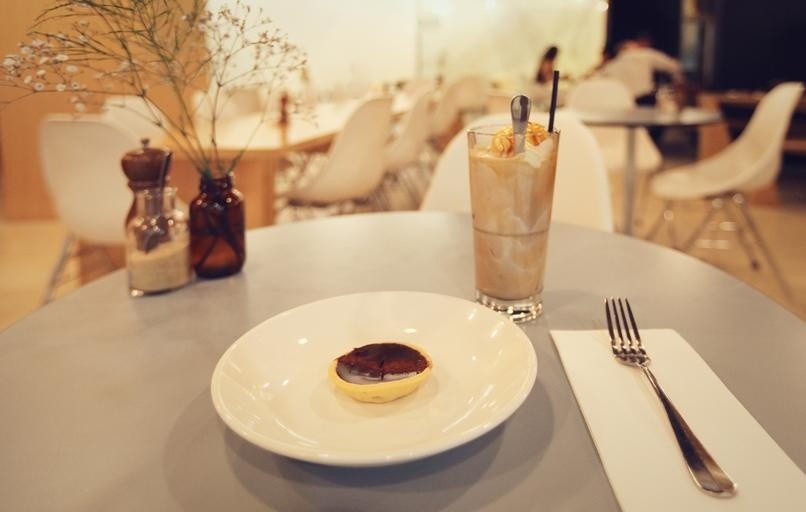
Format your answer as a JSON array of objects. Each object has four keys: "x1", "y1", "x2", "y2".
[{"x1": 578, "y1": 104, "x2": 720, "y2": 233}]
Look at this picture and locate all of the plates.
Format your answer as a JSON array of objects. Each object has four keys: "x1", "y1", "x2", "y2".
[{"x1": 210, "y1": 291, "x2": 538, "y2": 470}]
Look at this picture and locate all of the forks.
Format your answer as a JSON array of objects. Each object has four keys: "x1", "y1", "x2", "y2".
[{"x1": 605, "y1": 297, "x2": 738, "y2": 498}]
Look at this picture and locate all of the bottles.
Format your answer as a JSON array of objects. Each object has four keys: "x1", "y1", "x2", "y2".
[
  {"x1": 126, "y1": 189, "x2": 189, "y2": 294},
  {"x1": 120, "y1": 139, "x2": 172, "y2": 226}
]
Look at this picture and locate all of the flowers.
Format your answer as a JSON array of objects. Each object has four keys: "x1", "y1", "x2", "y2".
[{"x1": 0, "y1": 0, "x2": 320, "y2": 175}]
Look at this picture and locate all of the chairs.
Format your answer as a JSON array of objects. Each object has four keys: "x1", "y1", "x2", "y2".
[
  {"x1": 644, "y1": 82, "x2": 804, "y2": 299},
  {"x1": 419, "y1": 111, "x2": 613, "y2": 234},
  {"x1": 42, "y1": 113, "x2": 191, "y2": 302},
  {"x1": 565, "y1": 79, "x2": 663, "y2": 234},
  {"x1": 102, "y1": 96, "x2": 173, "y2": 144},
  {"x1": 289, "y1": 93, "x2": 399, "y2": 217},
  {"x1": 430, "y1": 80, "x2": 467, "y2": 150},
  {"x1": 385, "y1": 85, "x2": 438, "y2": 207}
]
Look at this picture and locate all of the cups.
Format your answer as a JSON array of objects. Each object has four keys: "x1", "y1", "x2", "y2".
[{"x1": 466, "y1": 123, "x2": 561, "y2": 325}]
